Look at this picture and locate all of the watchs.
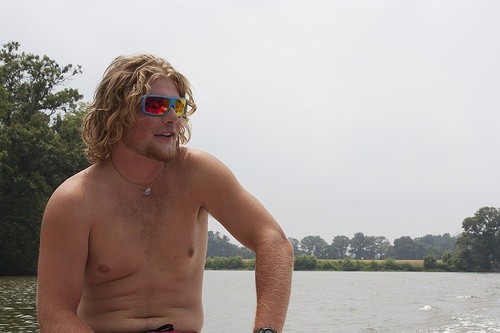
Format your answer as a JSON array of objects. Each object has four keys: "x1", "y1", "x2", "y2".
[{"x1": 253, "y1": 326, "x2": 277, "y2": 333}]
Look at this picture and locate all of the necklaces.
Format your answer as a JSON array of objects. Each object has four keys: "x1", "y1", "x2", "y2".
[{"x1": 110, "y1": 151, "x2": 165, "y2": 196}]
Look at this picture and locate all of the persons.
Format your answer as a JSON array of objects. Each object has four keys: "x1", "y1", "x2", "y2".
[{"x1": 37, "y1": 55, "x2": 294, "y2": 333}]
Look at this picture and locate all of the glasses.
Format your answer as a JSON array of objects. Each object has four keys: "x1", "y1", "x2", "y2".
[{"x1": 140, "y1": 94, "x2": 188, "y2": 119}]
